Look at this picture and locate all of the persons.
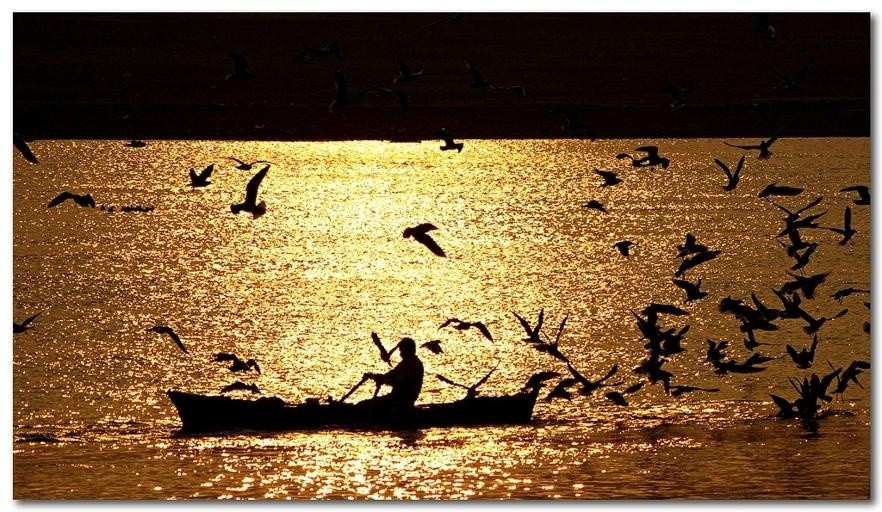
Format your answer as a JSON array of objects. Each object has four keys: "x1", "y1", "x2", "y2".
[{"x1": 356, "y1": 338, "x2": 424, "y2": 413}]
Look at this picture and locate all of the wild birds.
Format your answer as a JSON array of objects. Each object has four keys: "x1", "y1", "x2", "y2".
[
  {"x1": 438, "y1": 138, "x2": 464, "y2": 152},
  {"x1": 371, "y1": 332, "x2": 402, "y2": 367},
  {"x1": 14, "y1": 131, "x2": 42, "y2": 166},
  {"x1": 418, "y1": 307, "x2": 578, "y2": 401},
  {"x1": 13, "y1": 309, "x2": 45, "y2": 335},
  {"x1": 211, "y1": 352, "x2": 262, "y2": 397},
  {"x1": 568, "y1": 138, "x2": 872, "y2": 441},
  {"x1": 47, "y1": 191, "x2": 96, "y2": 208},
  {"x1": 187, "y1": 154, "x2": 273, "y2": 219},
  {"x1": 403, "y1": 223, "x2": 448, "y2": 258},
  {"x1": 145, "y1": 325, "x2": 189, "y2": 355}
]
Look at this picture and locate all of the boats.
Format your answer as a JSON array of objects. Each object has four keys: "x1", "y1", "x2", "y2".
[{"x1": 166, "y1": 388, "x2": 543, "y2": 428}]
